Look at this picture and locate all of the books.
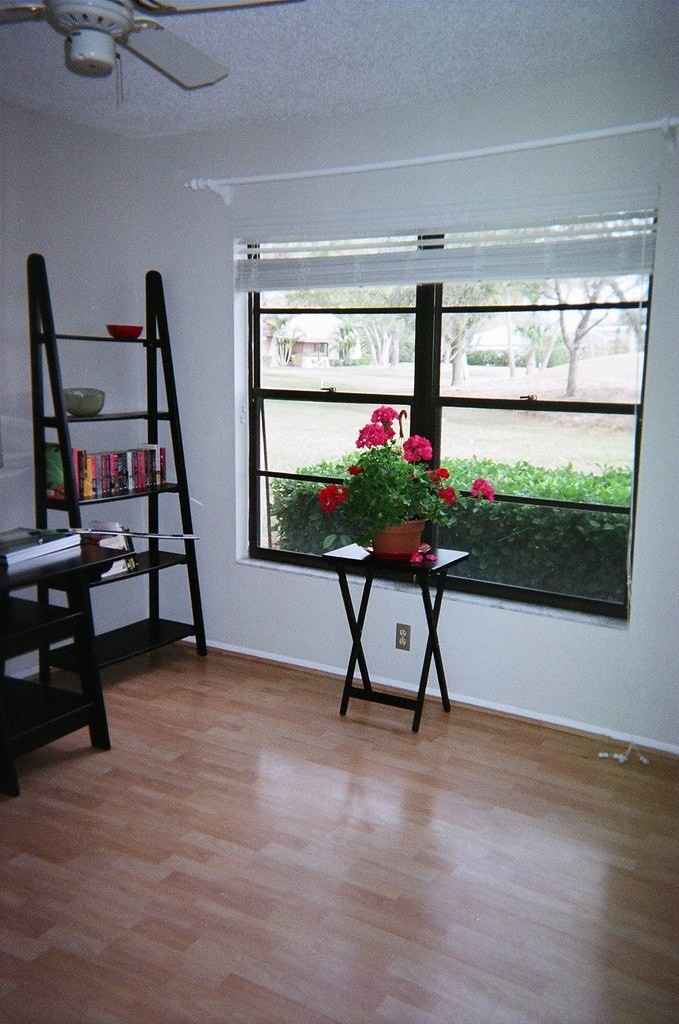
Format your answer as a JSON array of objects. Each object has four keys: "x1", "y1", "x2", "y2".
[
  {"x1": 0, "y1": 527, "x2": 81, "y2": 565},
  {"x1": 89, "y1": 520, "x2": 139, "y2": 578},
  {"x1": 46, "y1": 442, "x2": 166, "y2": 501}
]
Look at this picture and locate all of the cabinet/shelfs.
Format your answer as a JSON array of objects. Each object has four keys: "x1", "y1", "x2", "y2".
[
  {"x1": 27, "y1": 253, "x2": 208, "y2": 693},
  {"x1": 0, "y1": 530, "x2": 136, "y2": 798}
]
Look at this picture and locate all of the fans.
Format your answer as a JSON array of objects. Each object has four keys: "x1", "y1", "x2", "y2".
[{"x1": 0, "y1": 0, "x2": 302, "y2": 92}]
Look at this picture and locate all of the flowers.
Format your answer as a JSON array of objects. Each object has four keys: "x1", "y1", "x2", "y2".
[
  {"x1": 318, "y1": 406, "x2": 494, "y2": 547},
  {"x1": 409, "y1": 543, "x2": 437, "y2": 575}
]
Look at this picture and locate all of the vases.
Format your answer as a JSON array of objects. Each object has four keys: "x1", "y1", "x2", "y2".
[{"x1": 370, "y1": 511, "x2": 427, "y2": 560}]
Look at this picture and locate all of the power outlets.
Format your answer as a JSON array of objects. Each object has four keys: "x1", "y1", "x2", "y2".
[{"x1": 396, "y1": 623, "x2": 412, "y2": 653}]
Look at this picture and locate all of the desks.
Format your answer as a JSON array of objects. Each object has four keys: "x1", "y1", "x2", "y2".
[{"x1": 321, "y1": 537, "x2": 469, "y2": 734}]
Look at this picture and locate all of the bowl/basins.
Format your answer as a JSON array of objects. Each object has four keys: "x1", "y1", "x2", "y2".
[
  {"x1": 63, "y1": 388, "x2": 105, "y2": 417},
  {"x1": 106, "y1": 325, "x2": 143, "y2": 339}
]
